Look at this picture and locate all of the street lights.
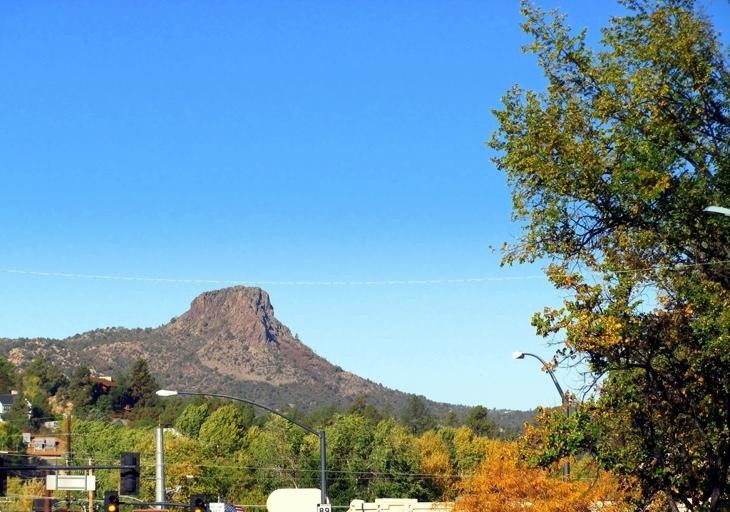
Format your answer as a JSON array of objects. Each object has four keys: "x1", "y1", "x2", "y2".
[
  {"x1": 513, "y1": 352, "x2": 572, "y2": 481},
  {"x1": 155, "y1": 389, "x2": 327, "y2": 505}
]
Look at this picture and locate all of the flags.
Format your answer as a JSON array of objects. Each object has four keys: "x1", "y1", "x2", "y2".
[{"x1": 225, "y1": 503, "x2": 243, "y2": 512}]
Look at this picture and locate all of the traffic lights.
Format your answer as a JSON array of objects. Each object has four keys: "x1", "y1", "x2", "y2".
[
  {"x1": 190, "y1": 493, "x2": 207, "y2": 512},
  {"x1": 105, "y1": 491, "x2": 119, "y2": 511}
]
